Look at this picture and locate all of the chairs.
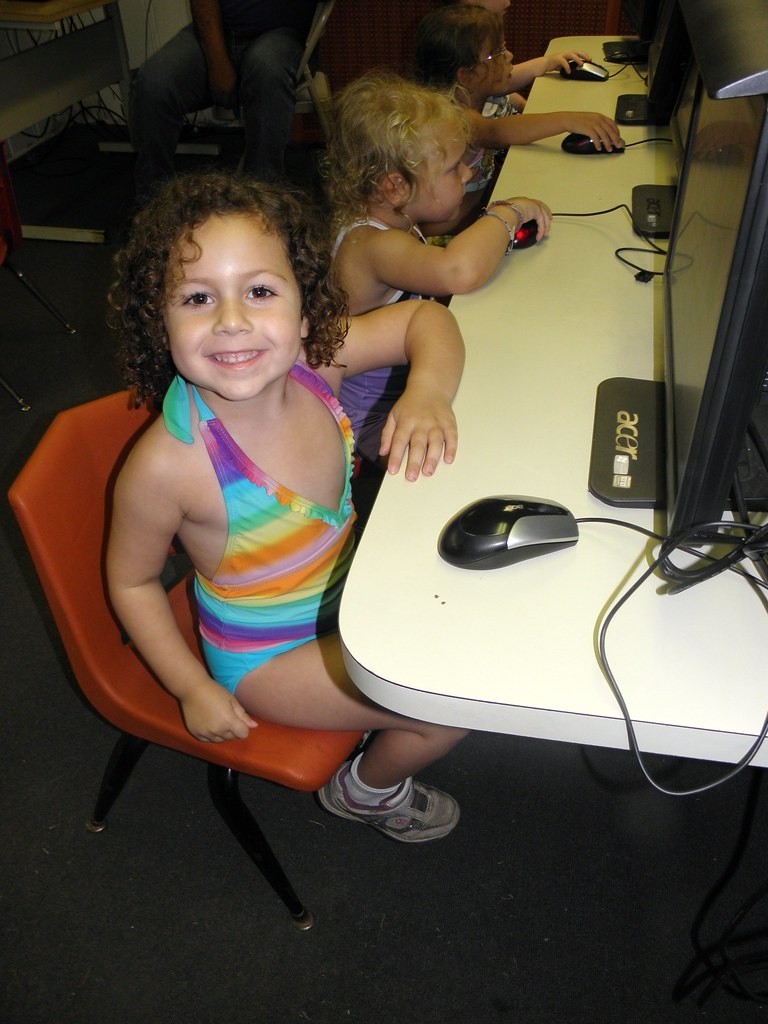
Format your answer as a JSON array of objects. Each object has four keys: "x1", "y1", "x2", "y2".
[
  {"x1": 0, "y1": 140, "x2": 76, "y2": 337},
  {"x1": 6, "y1": 385, "x2": 366, "y2": 930},
  {"x1": 209, "y1": 0, "x2": 336, "y2": 176}
]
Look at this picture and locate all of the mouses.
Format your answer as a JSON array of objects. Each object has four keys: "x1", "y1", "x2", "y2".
[
  {"x1": 561, "y1": 132, "x2": 625, "y2": 155},
  {"x1": 560, "y1": 60, "x2": 609, "y2": 82},
  {"x1": 438, "y1": 494, "x2": 579, "y2": 570},
  {"x1": 511, "y1": 219, "x2": 538, "y2": 249}
]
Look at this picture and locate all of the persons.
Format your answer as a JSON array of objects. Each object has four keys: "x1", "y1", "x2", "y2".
[
  {"x1": 312, "y1": 69, "x2": 555, "y2": 474},
  {"x1": 104, "y1": 168, "x2": 475, "y2": 847},
  {"x1": 126, "y1": 0, "x2": 321, "y2": 219},
  {"x1": 413, "y1": 0, "x2": 625, "y2": 203}
]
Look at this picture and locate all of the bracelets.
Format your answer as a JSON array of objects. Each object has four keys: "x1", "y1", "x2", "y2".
[
  {"x1": 489, "y1": 200, "x2": 523, "y2": 233},
  {"x1": 481, "y1": 208, "x2": 514, "y2": 257}
]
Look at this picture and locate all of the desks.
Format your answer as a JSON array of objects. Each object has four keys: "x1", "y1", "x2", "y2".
[{"x1": 337, "y1": 36, "x2": 768, "y2": 770}]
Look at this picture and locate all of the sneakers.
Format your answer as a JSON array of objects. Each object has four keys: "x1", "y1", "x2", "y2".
[{"x1": 317, "y1": 762, "x2": 462, "y2": 842}]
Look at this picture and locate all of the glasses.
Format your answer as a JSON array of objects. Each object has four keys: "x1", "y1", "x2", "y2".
[{"x1": 473, "y1": 46, "x2": 507, "y2": 66}]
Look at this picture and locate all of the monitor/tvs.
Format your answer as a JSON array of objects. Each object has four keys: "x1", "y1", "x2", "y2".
[{"x1": 588, "y1": 0, "x2": 768, "y2": 548}]
